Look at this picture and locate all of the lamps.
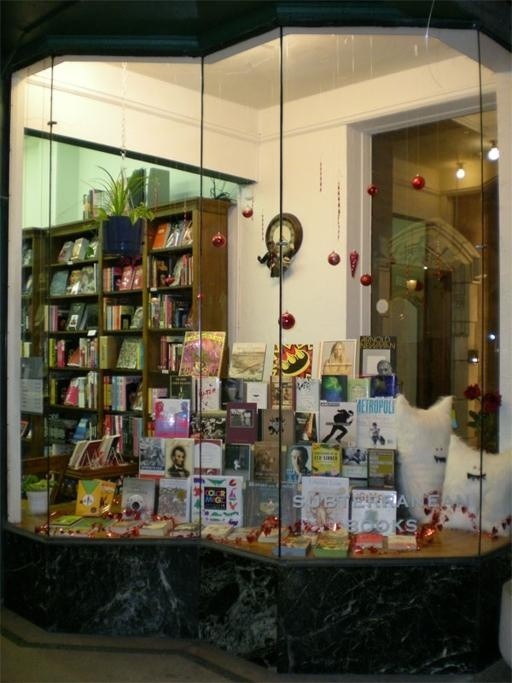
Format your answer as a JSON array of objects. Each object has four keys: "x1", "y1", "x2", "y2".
[
  {"x1": 489, "y1": 140, "x2": 500, "y2": 162},
  {"x1": 457, "y1": 162, "x2": 464, "y2": 178}
]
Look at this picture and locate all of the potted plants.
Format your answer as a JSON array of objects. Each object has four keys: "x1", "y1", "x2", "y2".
[{"x1": 85, "y1": 162, "x2": 156, "y2": 255}]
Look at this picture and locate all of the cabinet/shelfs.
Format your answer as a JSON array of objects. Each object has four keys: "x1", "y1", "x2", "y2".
[{"x1": 22, "y1": 196, "x2": 232, "y2": 462}]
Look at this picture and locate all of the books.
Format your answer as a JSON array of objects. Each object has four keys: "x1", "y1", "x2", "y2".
[
  {"x1": 83, "y1": 168, "x2": 170, "y2": 220},
  {"x1": 21, "y1": 212, "x2": 193, "y2": 538},
  {"x1": 179, "y1": 332, "x2": 418, "y2": 558}
]
